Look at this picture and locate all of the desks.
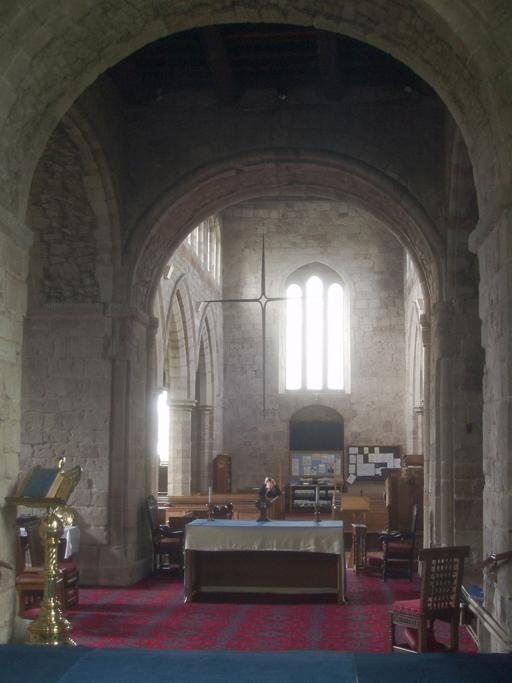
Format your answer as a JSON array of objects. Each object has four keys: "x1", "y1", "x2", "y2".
[{"x1": 183, "y1": 518, "x2": 345, "y2": 605}]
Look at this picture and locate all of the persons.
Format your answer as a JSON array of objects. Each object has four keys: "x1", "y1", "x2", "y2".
[{"x1": 257, "y1": 476, "x2": 282, "y2": 498}]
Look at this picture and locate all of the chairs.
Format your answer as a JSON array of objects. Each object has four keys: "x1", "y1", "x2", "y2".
[
  {"x1": 16, "y1": 517, "x2": 81, "y2": 620},
  {"x1": 386, "y1": 544, "x2": 471, "y2": 654},
  {"x1": 146, "y1": 494, "x2": 184, "y2": 577},
  {"x1": 377, "y1": 502, "x2": 418, "y2": 581}
]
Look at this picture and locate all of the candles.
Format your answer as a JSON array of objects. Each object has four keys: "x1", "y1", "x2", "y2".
[
  {"x1": 208, "y1": 486, "x2": 213, "y2": 505},
  {"x1": 315, "y1": 486, "x2": 319, "y2": 503}
]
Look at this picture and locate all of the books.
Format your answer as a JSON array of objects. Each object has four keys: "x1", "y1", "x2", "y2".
[
  {"x1": 320, "y1": 487, "x2": 340, "y2": 494},
  {"x1": 317, "y1": 481, "x2": 336, "y2": 485},
  {"x1": 300, "y1": 475, "x2": 317, "y2": 480},
  {"x1": 296, "y1": 480, "x2": 317, "y2": 484},
  {"x1": 294, "y1": 487, "x2": 315, "y2": 494},
  {"x1": 317, "y1": 477, "x2": 336, "y2": 482},
  {"x1": 22, "y1": 465, "x2": 62, "y2": 499}
]
